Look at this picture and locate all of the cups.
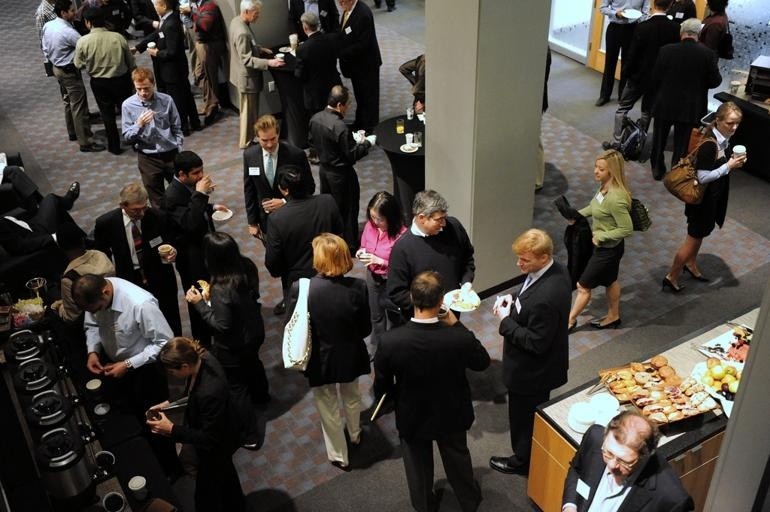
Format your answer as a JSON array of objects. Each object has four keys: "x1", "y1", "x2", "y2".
[
  {"x1": 396, "y1": 119, "x2": 405, "y2": 134},
  {"x1": 406, "y1": 105, "x2": 415, "y2": 120},
  {"x1": 102, "y1": 491, "x2": 125, "y2": 511},
  {"x1": 732, "y1": 144, "x2": 746, "y2": 159},
  {"x1": 261, "y1": 198, "x2": 272, "y2": 213},
  {"x1": 85, "y1": 379, "x2": 102, "y2": 391},
  {"x1": 145, "y1": 409, "x2": 162, "y2": 421},
  {"x1": 158, "y1": 244, "x2": 172, "y2": 263},
  {"x1": 289, "y1": 35, "x2": 297, "y2": 48},
  {"x1": 356, "y1": 130, "x2": 365, "y2": 142},
  {"x1": 413, "y1": 132, "x2": 422, "y2": 145},
  {"x1": 436, "y1": 304, "x2": 449, "y2": 317},
  {"x1": 405, "y1": 133, "x2": 413, "y2": 148},
  {"x1": 96, "y1": 450, "x2": 117, "y2": 468},
  {"x1": 276, "y1": 54, "x2": 284, "y2": 59},
  {"x1": 129, "y1": 476, "x2": 147, "y2": 499},
  {"x1": 147, "y1": 42, "x2": 156, "y2": 49},
  {"x1": 93, "y1": 403, "x2": 110, "y2": 416}
]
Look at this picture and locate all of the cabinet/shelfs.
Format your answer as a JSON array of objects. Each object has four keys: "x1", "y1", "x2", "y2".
[{"x1": 527, "y1": 306, "x2": 760, "y2": 512}]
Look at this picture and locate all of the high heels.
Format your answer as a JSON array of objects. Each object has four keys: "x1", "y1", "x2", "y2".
[
  {"x1": 683, "y1": 264, "x2": 706, "y2": 282},
  {"x1": 568, "y1": 320, "x2": 578, "y2": 333},
  {"x1": 662, "y1": 276, "x2": 686, "y2": 294},
  {"x1": 590, "y1": 318, "x2": 621, "y2": 329}
]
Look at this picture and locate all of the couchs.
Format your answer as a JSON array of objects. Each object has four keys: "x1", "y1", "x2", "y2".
[{"x1": 0, "y1": 207, "x2": 59, "y2": 286}]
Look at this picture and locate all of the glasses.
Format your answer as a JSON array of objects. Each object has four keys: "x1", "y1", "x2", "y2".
[{"x1": 599, "y1": 447, "x2": 640, "y2": 468}]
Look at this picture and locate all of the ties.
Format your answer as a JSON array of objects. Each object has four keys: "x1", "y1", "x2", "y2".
[
  {"x1": 266, "y1": 153, "x2": 275, "y2": 189},
  {"x1": 342, "y1": 11, "x2": 348, "y2": 31},
  {"x1": 131, "y1": 221, "x2": 142, "y2": 268}
]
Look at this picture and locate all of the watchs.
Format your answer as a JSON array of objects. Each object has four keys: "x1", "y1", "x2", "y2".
[{"x1": 124, "y1": 358, "x2": 133, "y2": 371}]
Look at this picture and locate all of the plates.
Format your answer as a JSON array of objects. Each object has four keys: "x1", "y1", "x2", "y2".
[
  {"x1": 212, "y1": 208, "x2": 232, "y2": 222},
  {"x1": 279, "y1": 47, "x2": 293, "y2": 52},
  {"x1": 621, "y1": 9, "x2": 642, "y2": 20},
  {"x1": 598, "y1": 362, "x2": 680, "y2": 402},
  {"x1": 698, "y1": 326, "x2": 753, "y2": 361},
  {"x1": 399, "y1": 143, "x2": 419, "y2": 154},
  {"x1": 692, "y1": 359, "x2": 746, "y2": 399},
  {"x1": 567, "y1": 412, "x2": 594, "y2": 434},
  {"x1": 444, "y1": 288, "x2": 481, "y2": 313},
  {"x1": 628, "y1": 376, "x2": 722, "y2": 426}
]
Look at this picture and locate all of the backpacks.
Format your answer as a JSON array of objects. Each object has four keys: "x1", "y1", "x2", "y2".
[
  {"x1": 721, "y1": 20, "x2": 735, "y2": 59},
  {"x1": 283, "y1": 278, "x2": 313, "y2": 372},
  {"x1": 618, "y1": 116, "x2": 649, "y2": 160}
]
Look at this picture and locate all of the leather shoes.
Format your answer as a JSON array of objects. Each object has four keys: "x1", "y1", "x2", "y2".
[
  {"x1": 489, "y1": 454, "x2": 530, "y2": 478},
  {"x1": 64, "y1": 181, "x2": 80, "y2": 211},
  {"x1": 80, "y1": 141, "x2": 106, "y2": 152},
  {"x1": 594, "y1": 95, "x2": 612, "y2": 109},
  {"x1": 273, "y1": 299, "x2": 286, "y2": 315}
]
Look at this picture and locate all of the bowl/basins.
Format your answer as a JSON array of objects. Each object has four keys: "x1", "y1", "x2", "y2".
[
  {"x1": 570, "y1": 401, "x2": 598, "y2": 424},
  {"x1": 593, "y1": 393, "x2": 620, "y2": 424}
]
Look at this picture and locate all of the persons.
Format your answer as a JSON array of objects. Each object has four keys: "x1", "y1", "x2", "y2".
[
  {"x1": 229, "y1": 0, "x2": 286, "y2": 150},
  {"x1": 129, "y1": 0, "x2": 203, "y2": 136},
  {"x1": 181, "y1": 0, "x2": 230, "y2": 110},
  {"x1": 356, "y1": 192, "x2": 407, "y2": 361},
  {"x1": 384, "y1": 189, "x2": 476, "y2": 328},
  {"x1": 601, "y1": 0, "x2": 681, "y2": 155},
  {"x1": 596, "y1": 0, "x2": 651, "y2": 107},
  {"x1": 73, "y1": 7, "x2": 137, "y2": 155},
  {"x1": 651, "y1": 18, "x2": 722, "y2": 181},
  {"x1": 0, "y1": 181, "x2": 81, "y2": 255},
  {"x1": 71, "y1": 274, "x2": 174, "y2": 435},
  {"x1": 535, "y1": 43, "x2": 552, "y2": 192},
  {"x1": 94, "y1": 183, "x2": 183, "y2": 337},
  {"x1": 490, "y1": 229, "x2": 573, "y2": 477},
  {"x1": 50, "y1": 222, "x2": 115, "y2": 379},
  {"x1": 71, "y1": 1, "x2": 136, "y2": 40},
  {"x1": 282, "y1": 233, "x2": 373, "y2": 468},
  {"x1": 39, "y1": 0, "x2": 106, "y2": 152},
  {"x1": 160, "y1": 151, "x2": 218, "y2": 344},
  {"x1": 663, "y1": 101, "x2": 747, "y2": 293},
  {"x1": 34, "y1": 0, "x2": 58, "y2": 46},
  {"x1": 145, "y1": 337, "x2": 247, "y2": 512},
  {"x1": 560, "y1": 411, "x2": 696, "y2": 511},
  {"x1": 308, "y1": 85, "x2": 378, "y2": 257},
  {"x1": 289, "y1": 13, "x2": 341, "y2": 163},
  {"x1": 178, "y1": 0, "x2": 224, "y2": 126},
  {"x1": 374, "y1": 271, "x2": 490, "y2": 512},
  {"x1": 290, "y1": 0, "x2": 339, "y2": 50},
  {"x1": 133, "y1": 0, "x2": 160, "y2": 38},
  {"x1": 568, "y1": 149, "x2": 633, "y2": 334},
  {"x1": 399, "y1": 55, "x2": 426, "y2": 116},
  {"x1": 336, "y1": 0, "x2": 382, "y2": 136},
  {"x1": 122, "y1": 68, "x2": 184, "y2": 209},
  {"x1": 667, "y1": 0, "x2": 697, "y2": 18},
  {"x1": 699, "y1": 0, "x2": 735, "y2": 60},
  {"x1": 185, "y1": 232, "x2": 271, "y2": 411},
  {"x1": 265, "y1": 164, "x2": 345, "y2": 316},
  {"x1": 243, "y1": 115, "x2": 315, "y2": 316},
  {"x1": 0, "y1": 165, "x2": 43, "y2": 213}
]
